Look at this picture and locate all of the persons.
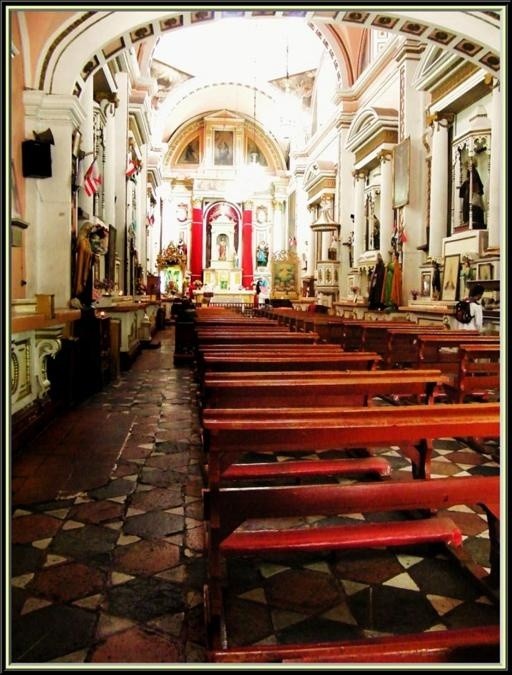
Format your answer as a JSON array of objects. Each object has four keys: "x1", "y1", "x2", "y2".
[
  {"x1": 451, "y1": 283, "x2": 486, "y2": 332},
  {"x1": 367, "y1": 252, "x2": 385, "y2": 310},
  {"x1": 459, "y1": 156, "x2": 486, "y2": 229},
  {"x1": 391, "y1": 252, "x2": 404, "y2": 307},
  {"x1": 383, "y1": 251, "x2": 394, "y2": 306},
  {"x1": 258, "y1": 241, "x2": 268, "y2": 266},
  {"x1": 217, "y1": 137, "x2": 231, "y2": 165},
  {"x1": 218, "y1": 237, "x2": 226, "y2": 261}
]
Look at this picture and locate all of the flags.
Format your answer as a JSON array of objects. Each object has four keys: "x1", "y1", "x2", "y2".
[
  {"x1": 125, "y1": 160, "x2": 137, "y2": 179},
  {"x1": 83, "y1": 156, "x2": 102, "y2": 197}
]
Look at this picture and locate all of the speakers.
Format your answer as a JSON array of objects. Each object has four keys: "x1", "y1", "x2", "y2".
[{"x1": 22, "y1": 139, "x2": 51, "y2": 177}]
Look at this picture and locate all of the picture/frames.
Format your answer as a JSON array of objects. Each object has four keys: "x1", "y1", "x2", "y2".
[
  {"x1": 392, "y1": 135, "x2": 410, "y2": 209},
  {"x1": 439, "y1": 254, "x2": 460, "y2": 302},
  {"x1": 478, "y1": 263, "x2": 493, "y2": 280}
]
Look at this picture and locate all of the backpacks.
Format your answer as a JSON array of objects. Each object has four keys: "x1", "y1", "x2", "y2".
[{"x1": 455, "y1": 298, "x2": 480, "y2": 323}]
